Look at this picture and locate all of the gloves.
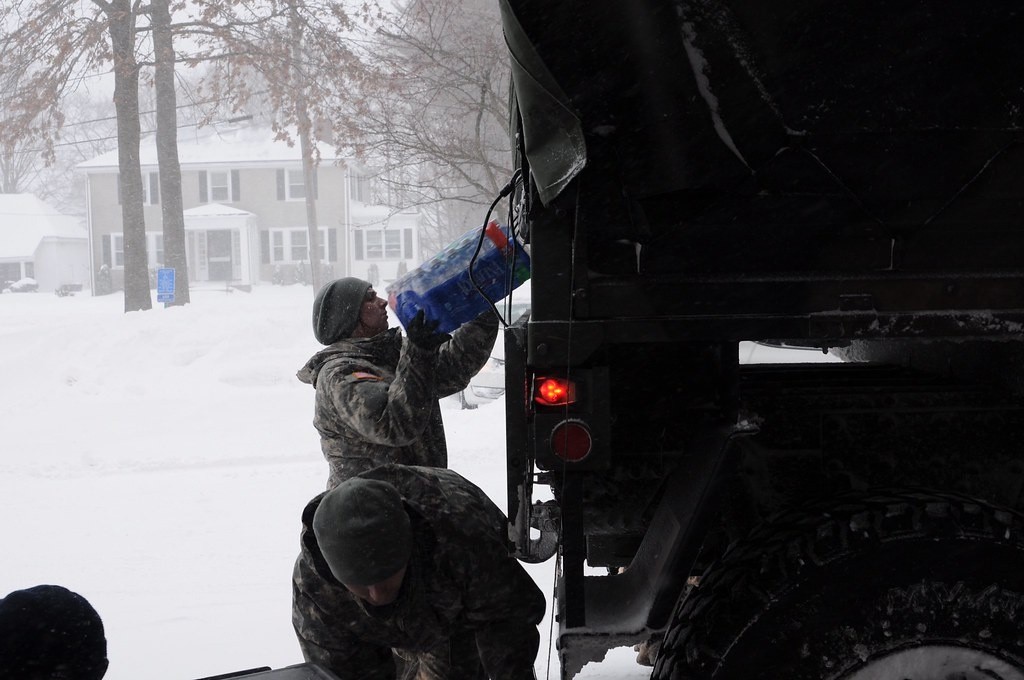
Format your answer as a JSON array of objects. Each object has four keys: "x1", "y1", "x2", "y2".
[{"x1": 407, "y1": 310, "x2": 453, "y2": 352}]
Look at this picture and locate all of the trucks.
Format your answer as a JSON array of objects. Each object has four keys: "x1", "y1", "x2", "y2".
[{"x1": 495, "y1": 0, "x2": 1024, "y2": 680}]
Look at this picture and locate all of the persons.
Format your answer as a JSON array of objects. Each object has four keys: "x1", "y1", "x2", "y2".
[
  {"x1": 298, "y1": 277, "x2": 499, "y2": 488},
  {"x1": 292, "y1": 464, "x2": 546, "y2": 680},
  {"x1": 0, "y1": 585, "x2": 109, "y2": 680}
]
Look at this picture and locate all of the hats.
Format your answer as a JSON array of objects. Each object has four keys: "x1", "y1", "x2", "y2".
[
  {"x1": 312, "y1": 277, "x2": 377, "y2": 345},
  {"x1": 313, "y1": 478, "x2": 413, "y2": 585}
]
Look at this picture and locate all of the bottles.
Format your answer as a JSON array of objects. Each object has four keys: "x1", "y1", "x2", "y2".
[{"x1": 386, "y1": 219, "x2": 530, "y2": 335}]
